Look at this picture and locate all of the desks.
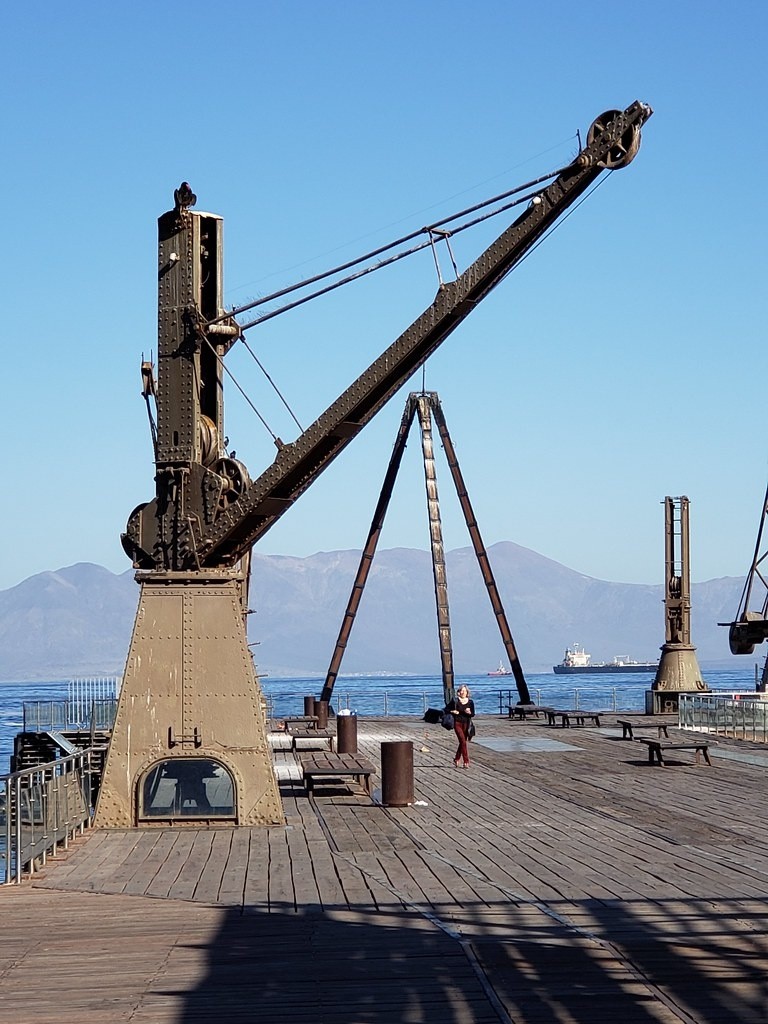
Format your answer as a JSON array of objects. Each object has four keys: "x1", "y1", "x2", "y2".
[
  {"x1": 282, "y1": 715, "x2": 319, "y2": 734},
  {"x1": 641, "y1": 740, "x2": 718, "y2": 766},
  {"x1": 617, "y1": 719, "x2": 674, "y2": 742},
  {"x1": 300, "y1": 754, "x2": 377, "y2": 801},
  {"x1": 289, "y1": 729, "x2": 337, "y2": 760},
  {"x1": 505, "y1": 705, "x2": 603, "y2": 728}
]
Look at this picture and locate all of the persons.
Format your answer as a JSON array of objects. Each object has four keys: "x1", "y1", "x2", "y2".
[{"x1": 444, "y1": 685, "x2": 475, "y2": 769}]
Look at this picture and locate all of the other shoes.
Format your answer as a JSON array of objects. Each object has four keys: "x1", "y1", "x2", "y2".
[
  {"x1": 453, "y1": 759, "x2": 459, "y2": 767},
  {"x1": 463, "y1": 763, "x2": 469, "y2": 769}
]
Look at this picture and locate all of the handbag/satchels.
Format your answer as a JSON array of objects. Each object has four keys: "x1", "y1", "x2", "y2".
[
  {"x1": 467, "y1": 721, "x2": 475, "y2": 741},
  {"x1": 441, "y1": 714, "x2": 454, "y2": 731}
]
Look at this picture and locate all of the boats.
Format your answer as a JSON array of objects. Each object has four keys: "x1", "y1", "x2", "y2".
[
  {"x1": 553, "y1": 643, "x2": 659, "y2": 674},
  {"x1": 488, "y1": 660, "x2": 513, "y2": 676}
]
